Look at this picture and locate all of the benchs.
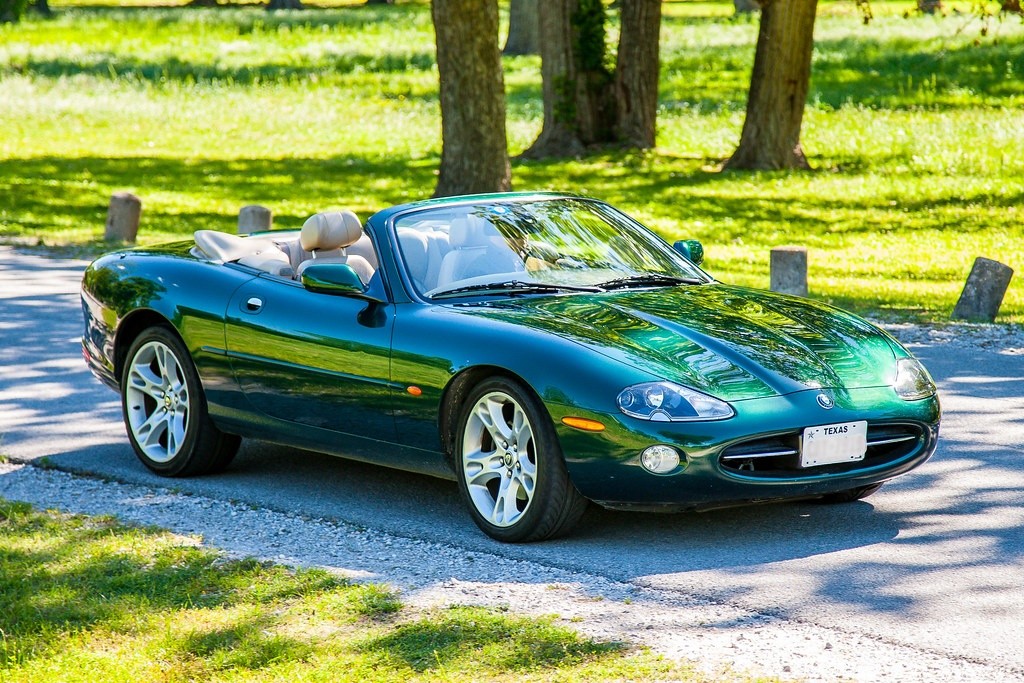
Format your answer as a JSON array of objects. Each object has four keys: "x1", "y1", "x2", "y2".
[{"x1": 195, "y1": 228, "x2": 449, "y2": 289}]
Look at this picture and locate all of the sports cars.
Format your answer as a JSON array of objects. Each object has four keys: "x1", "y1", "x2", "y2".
[{"x1": 79, "y1": 191, "x2": 943, "y2": 546}]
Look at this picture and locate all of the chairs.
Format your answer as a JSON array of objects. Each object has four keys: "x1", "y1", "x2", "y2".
[
  {"x1": 296, "y1": 211, "x2": 376, "y2": 285},
  {"x1": 439, "y1": 217, "x2": 526, "y2": 285}
]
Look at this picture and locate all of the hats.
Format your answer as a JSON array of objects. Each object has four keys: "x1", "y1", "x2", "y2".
[{"x1": 483, "y1": 202, "x2": 544, "y2": 237}]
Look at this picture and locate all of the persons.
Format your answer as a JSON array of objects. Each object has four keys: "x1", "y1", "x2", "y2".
[{"x1": 466, "y1": 206, "x2": 561, "y2": 279}]
